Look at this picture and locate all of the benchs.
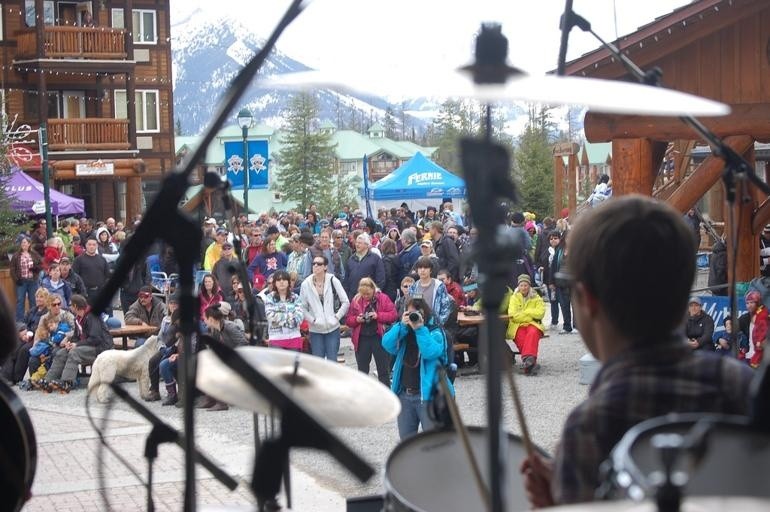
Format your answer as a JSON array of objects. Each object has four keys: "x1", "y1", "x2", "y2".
[
  {"x1": 452, "y1": 342, "x2": 470, "y2": 377},
  {"x1": 76, "y1": 359, "x2": 94, "y2": 386},
  {"x1": 541, "y1": 335, "x2": 549, "y2": 338}
]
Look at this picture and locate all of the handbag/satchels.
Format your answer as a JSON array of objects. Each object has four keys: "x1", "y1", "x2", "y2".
[
  {"x1": 427, "y1": 364, "x2": 457, "y2": 421},
  {"x1": 330, "y1": 276, "x2": 342, "y2": 314},
  {"x1": 541, "y1": 283, "x2": 558, "y2": 303}
]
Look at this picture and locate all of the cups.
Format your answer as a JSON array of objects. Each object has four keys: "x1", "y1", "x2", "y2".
[{"x1": 27, "y1": 330, "x2": 33, "y2": 342}]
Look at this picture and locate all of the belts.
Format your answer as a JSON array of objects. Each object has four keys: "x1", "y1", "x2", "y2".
[{"x1": 404, "y1": 388, "x2": 419, "y2": 396}]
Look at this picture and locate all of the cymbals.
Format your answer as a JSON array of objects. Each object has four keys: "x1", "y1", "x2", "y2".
[
  {"x1": 194, "y1": 345, "x2": 402, "y2": 427},
  {"x1": 254, "y1": 68, "x2": 733, "y2": 118}
]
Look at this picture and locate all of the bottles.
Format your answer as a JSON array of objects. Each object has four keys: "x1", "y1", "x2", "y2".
[
  {"x1": 550, "y1": 288, "x2": 557, "y2": 303},
  {"x1": 394, "y1": 288, "x2": 401, "y2": 305}
]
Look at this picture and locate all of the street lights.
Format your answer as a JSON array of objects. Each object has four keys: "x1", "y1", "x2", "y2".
[{"x1": 237, "y1": 108, "x2": 253, "y2": 212}]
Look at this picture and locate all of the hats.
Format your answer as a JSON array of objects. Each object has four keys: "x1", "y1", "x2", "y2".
[
  {"x1": 421, "y1": 240, "x2": 432, "y2": 248},
  {"x1": 138, "y1": 286, "x2": 152, "y2": 296},
  {"x1": 688, "y1": 297, "x2": 702, "y2": 306},
  {"x1": 206, "y1": 218, "x2": 216, "y2": 226},
  {"x1": 61, "y1": 257, "x2": 70, "y2": 264},
  {"x1": 517, "y1": 274, "x2": 532, "y2": 285},
  {"x1": 217, "y1": 227, "x2": 228, "y2": 236}
]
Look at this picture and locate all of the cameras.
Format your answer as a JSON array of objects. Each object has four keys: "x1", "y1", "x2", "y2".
[
  {"x1": 360, "y1": 312, "x2": 369, "y2": 320},
  {"x1": 405, "y1": 310, "x2": 422, "y2": 323}
]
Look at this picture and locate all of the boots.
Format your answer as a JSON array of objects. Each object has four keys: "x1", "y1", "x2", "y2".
[{"x1": 162, "y1": 380, "x2": 179, "y2": 406}]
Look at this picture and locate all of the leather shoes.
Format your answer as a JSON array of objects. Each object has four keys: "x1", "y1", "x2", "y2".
[
  {"x1": 207, "y1": 403, "x2": 228, "y2": 411},
  {"x1": 195, "y1": 398, "x2": 215, "y2": 409}
]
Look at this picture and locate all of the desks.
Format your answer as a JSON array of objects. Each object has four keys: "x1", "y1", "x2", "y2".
[
  {"x1": 108, "y1": 325, "x2": 158, "y2": 383},
  {"x1": 456, "y1": 311, "x2": 513, "y2": 376}
]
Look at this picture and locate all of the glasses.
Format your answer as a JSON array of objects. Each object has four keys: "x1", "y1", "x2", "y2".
[
  {"x1": 312, "y1": 262, "x2": 325, "y2": 266},
  {"x1": 554, "y1": 272, "x2": 579, "y2": 288},
  {"x1": 404, "y1": 285, "x2": 412, "y2": 288},
  {"x1": 52, "y1": 303, "x2": 61, "y2": 307},
  {"x1": 276, "y1": 277, "x2": 288, "y2": 282}
]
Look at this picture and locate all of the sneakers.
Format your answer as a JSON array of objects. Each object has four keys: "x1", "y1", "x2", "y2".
[
  {"x1": 38, "y1": 378, "x2": 52, "y2": 393},
  {"x1": 146, "y1": 392, "x2": 161, "y2": 401},
  {"x1": 528, "y1": 369, "x2": 537, "y2": 376},
  {"x1": 49, "y1": 379, "x2": 71, "y2": 393},
  {"x1": 520, "y1": 357, "x2": 536, "y2": 370},
  {"x1": 550, "y1": 324, "x2": 579, "y2": 335}
]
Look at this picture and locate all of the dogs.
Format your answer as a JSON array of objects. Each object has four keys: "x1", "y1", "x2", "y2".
[{"x1": 87, "y1": 334, "x2": 166, "y2": 403}]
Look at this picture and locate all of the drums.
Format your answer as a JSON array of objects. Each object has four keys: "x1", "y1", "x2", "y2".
[
  {"x1": 592, "y1": 413, "x2": 770, "y2": 502},
  {"x1": 384, "y1": 423, "x2": 553, "y2": 512}
]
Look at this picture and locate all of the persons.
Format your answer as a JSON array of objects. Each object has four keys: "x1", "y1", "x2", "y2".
[
  {"x1": 79, "y1": 11, "x2": 99, "y2": 60},
  {"x1": 583, "y1": 174, "x2": 615, "y2": 211},
  {"x1": 677, "y1": 207, "x2": 770, "y2": 370},
  {"x1": 7, "y1": 201, "x2": 572, "y2": 413},
  {"x1": 380, "y1": 298, "x2": 456, "y2": 443},
  {"x1": 516, "y1": 193, "x2": 770, "y2": 512}
]
